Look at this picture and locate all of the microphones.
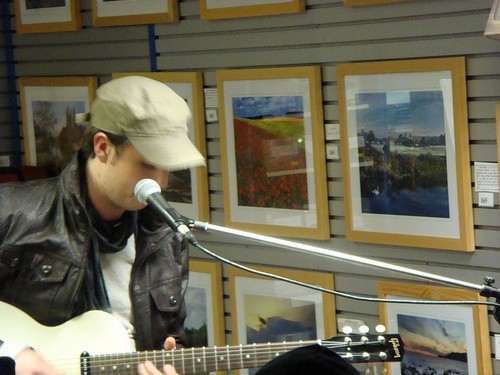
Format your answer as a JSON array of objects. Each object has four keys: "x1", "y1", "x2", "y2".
[{"x1": 134, "y1": 177, "x2": 199, "y2": 246}]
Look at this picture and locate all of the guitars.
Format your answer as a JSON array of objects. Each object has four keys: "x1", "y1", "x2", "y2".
[{"x1": 0, "y1": 299, "x2": 405, "y2": 375}]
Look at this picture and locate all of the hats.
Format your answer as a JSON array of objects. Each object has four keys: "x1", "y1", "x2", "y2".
[{"x1": 73, "y1": 75, "x2": 207, "y2": 172}]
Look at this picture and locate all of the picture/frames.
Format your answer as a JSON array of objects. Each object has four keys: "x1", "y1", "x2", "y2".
[
  {"x1": 175, "y1": 256, "x2": 227, "y2": 375},
  {"x1": 377, "y1": 281, "x2": 494, "y2": 374},
  {"x1": 199, "y1": 0, "x2": 307, "y2": 21},
  {"x1": 228, "y1": 263, "x2": 337, "y2": 375},
  {"x1": 91, "y1": 0, "x2": 180, "y2": 29},
  {"x1": 15, "y1": 0, "x2": 82, "y2": 36},
  {"x1": 20, "y1": 75, "x2": 99, "y2": 169},
  {"x1": 217, "y1": 63, "x2": 331, "y2": 242},
  {"x1": 112, "y1": 70, "x2": 211, "y2": 224},
  {"x1": 337, "y1": 56, "x2": 476, "y2": 253}
]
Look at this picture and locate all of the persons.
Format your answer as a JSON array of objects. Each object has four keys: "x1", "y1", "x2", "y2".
[{"x1": 0, "y1": 76, "x2": 206, "y2": 375}]
